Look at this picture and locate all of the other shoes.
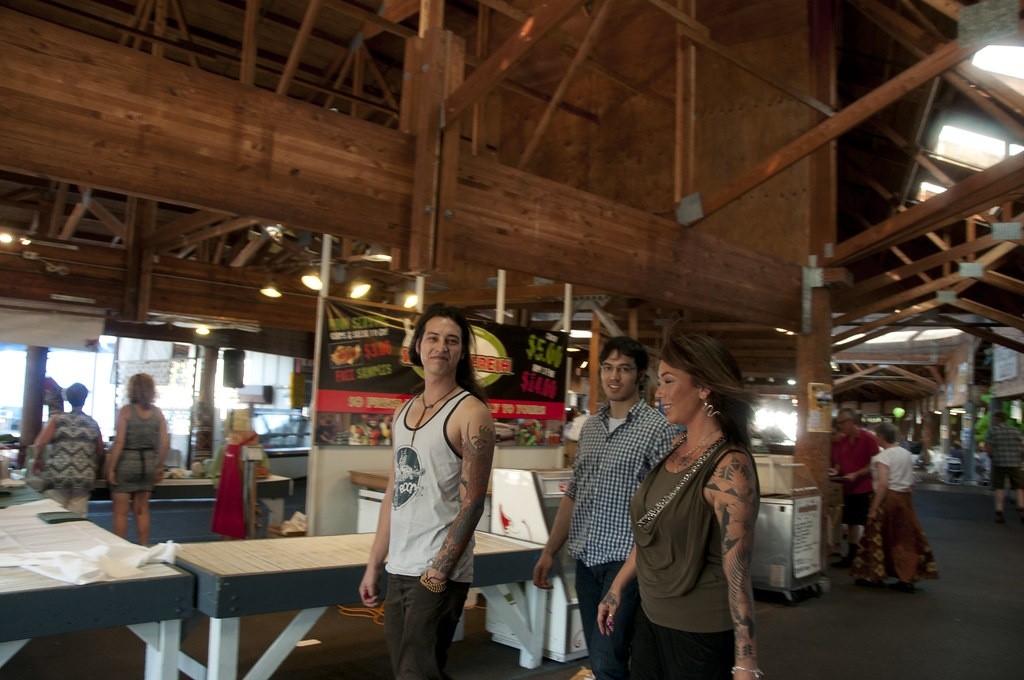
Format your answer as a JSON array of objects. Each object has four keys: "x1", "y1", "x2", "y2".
[
  {"x1": 854, "y1": 577, "x2": 886, "y2": 589},
  {"x1": 830, "y1": 558, "x2": 853, "y2": 570},
  {"x1": 890, "y1": 581, "x2": 916, "y2": 593},
  {"x1": 995, "y1": 513, "x2": 1007, "y2": 524}
]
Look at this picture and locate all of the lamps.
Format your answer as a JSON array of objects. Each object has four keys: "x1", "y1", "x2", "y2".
[
  {"x1": 259, "y1": 277, "x2": 283, "y2": 298},
  {"x1": 345, "y1": 277, "x2": 372, "y2": 300},
  {"x1": 395, "y1": 288, "x2": 420, "y2": 309},
  {"x1": 300, "y1": 263, "x2": 324, "y2": 291}
]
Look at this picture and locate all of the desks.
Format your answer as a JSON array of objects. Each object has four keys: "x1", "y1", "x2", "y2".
[
  {"x1": 154, "y1": 527, "x2": 561, "y2": 680},
  {"x1": 0, "y1": 473, "x2": 195, "y2": 680},
  {"x1": 88, "y1": 472, "x2": 294, "y2": 502}
]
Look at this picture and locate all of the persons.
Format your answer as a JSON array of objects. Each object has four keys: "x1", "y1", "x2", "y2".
[
  {"x1": 533, "y1": 336, "x2": 683, "y2": 680},
  {"x1": 985, "y1": 411, "x2": 1024, "y2": 524},
  {"x1": 827, "y1": 408, "x2": 880, "y2": 569},
  {"x1": 106, "y1": 373, "x2": 170, "y2": 547},
  {"x1": 32, "y1": 383, "x2": 107, "y2": 519},
  {"x1": 360, "y1": 301, "x2": 496, "y2": 680},
  {"x1": 597, "y1": 336, "x2": 764, "y2": 680},
  {"x1": 849, "y1": 421, "x2": 940, "y2": 594}
]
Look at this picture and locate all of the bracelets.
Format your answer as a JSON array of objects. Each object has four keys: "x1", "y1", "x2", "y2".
[
  {"x1": 419, "y1": 570, "x2": 447, "y2": 593},
  {"x1": 731, "y1": 665, "x2": 764, "y2": 677}
]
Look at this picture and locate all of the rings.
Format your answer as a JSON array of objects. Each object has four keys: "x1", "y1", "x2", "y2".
[{"x1": 608, "y1": 614, "x2": 615, "y2": 617}]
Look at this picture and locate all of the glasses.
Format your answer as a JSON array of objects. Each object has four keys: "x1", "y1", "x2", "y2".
[{"x1": 835, "y1": 418, "x2": 851, "y2": 425}]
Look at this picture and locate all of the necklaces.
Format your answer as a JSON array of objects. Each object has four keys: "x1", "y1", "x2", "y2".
[
  {"x1": 673, "y1": 428, "x2": 720, "y2": 475},
  {"x1": 410, "y1": 383, "x2": 457, "y2": 448}
]
